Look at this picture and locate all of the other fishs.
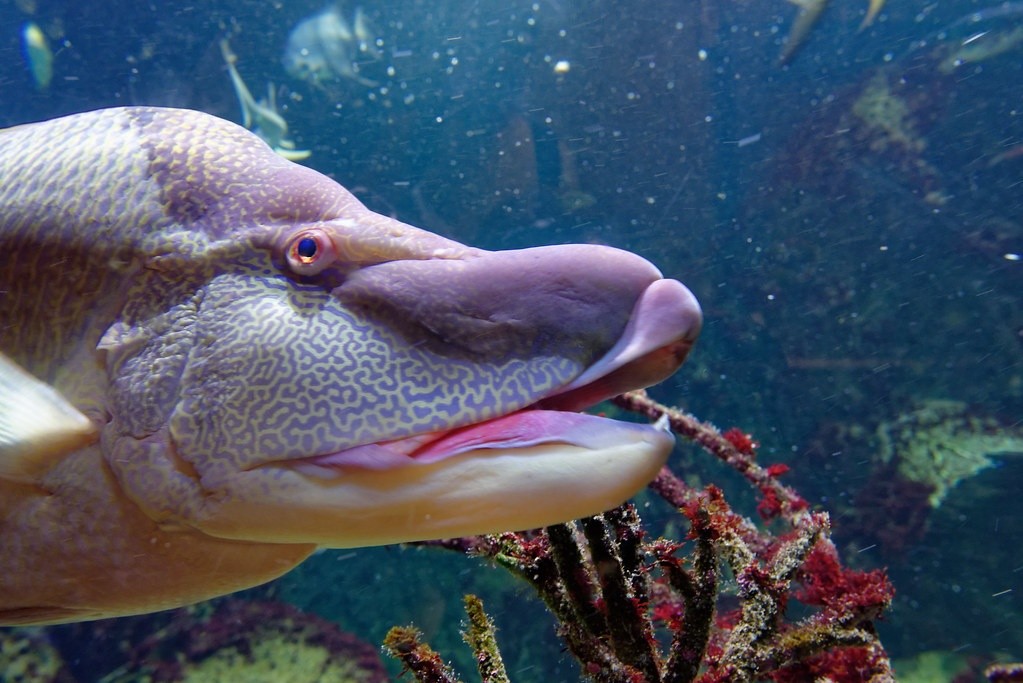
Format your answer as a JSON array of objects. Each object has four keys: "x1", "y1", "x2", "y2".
[{"x1": 1, "y1": 103, "x2": 704, "y2": 630}]
